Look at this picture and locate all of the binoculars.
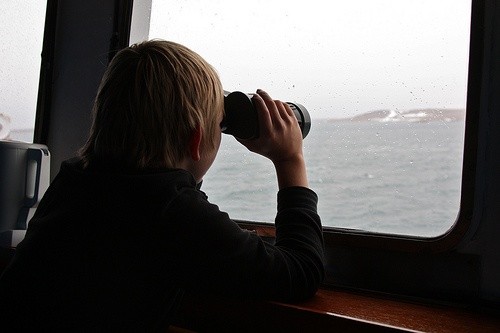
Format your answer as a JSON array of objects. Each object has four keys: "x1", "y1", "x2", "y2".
[{"x1": 222, "y1": 90, "x2": 311, "y2": 142}]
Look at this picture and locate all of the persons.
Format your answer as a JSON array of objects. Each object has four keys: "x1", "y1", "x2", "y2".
[{"x1": 0, "y1": 40, "x2": 326, "y2": 333}]
